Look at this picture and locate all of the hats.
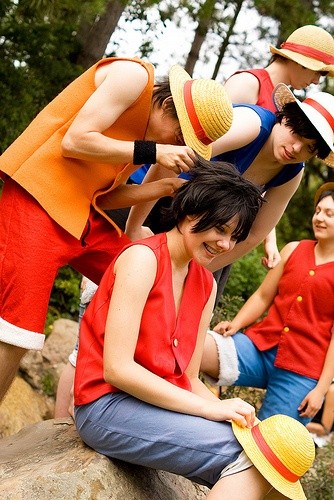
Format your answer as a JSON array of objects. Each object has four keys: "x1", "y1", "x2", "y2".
[
  {"x1": 269, "y1": 25, "x2": 334, "y2": 77},
  {"x1": 314, "y1": 183, "x2": 334, "y2": 207},
  {"x1": 169, "y1": 63, "x2": 233, "y2": 161},
  {"x1": 231, "y1": 414, "x2": 315, "y2": 500},
  {"x1": 272, "y1": 83, "x2": 334, "y2": 168}
]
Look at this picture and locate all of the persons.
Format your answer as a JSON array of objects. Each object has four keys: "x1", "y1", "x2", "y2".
[
  {"x1": 74, "y1": 161, "x2": 317, "y2": 500},
  {"x1": 99, "y1": 81, "x2": 333, "y2": 275},
  {"x1": 206, "y1": 24, "x2": 334, "y2": 325},
  {"x1": 198, "y1": 181, "x2": 334, "y2": 434},
  {"x1": 1, "y1": 58, "x2": 233, "y2": 403}
]
